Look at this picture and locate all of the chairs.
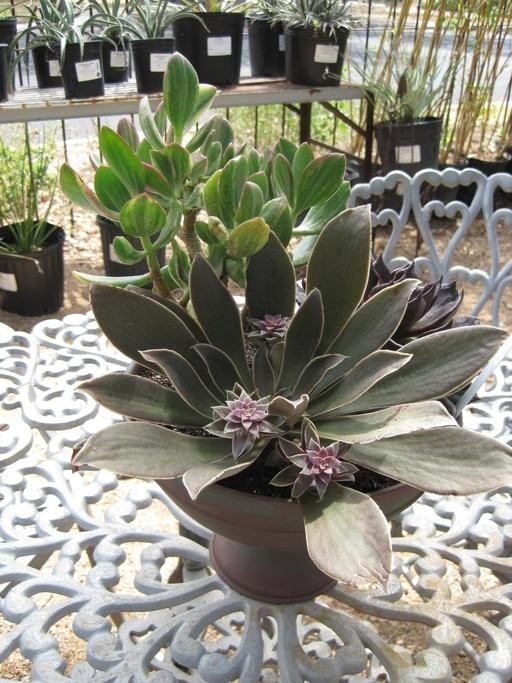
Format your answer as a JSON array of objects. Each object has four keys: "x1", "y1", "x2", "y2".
[{"x1": 335, "y1": 168, "x2": 512, "y2": 337}]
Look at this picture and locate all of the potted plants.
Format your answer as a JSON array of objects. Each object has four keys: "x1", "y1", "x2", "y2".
[
  {"x1": 55, "y1": 48, "x2": 511, "y2": 604},
  {"x1": 0, "y1": 119, "x2": 72, "y2": 318},
  {"x1": 327, "y1": 26, "x2": 478, "y2": 172},
  {"x1": 2, "y1": 0, "x2": 352, "y2": 102}
]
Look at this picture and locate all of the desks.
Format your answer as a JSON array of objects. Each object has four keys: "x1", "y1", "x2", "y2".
[
  {"x1": 0, "y1": 68, "x2": 377, "y2": 185},
  {"x1": 0, "y1": 306, "x2": 512, "y2": 681}
]
